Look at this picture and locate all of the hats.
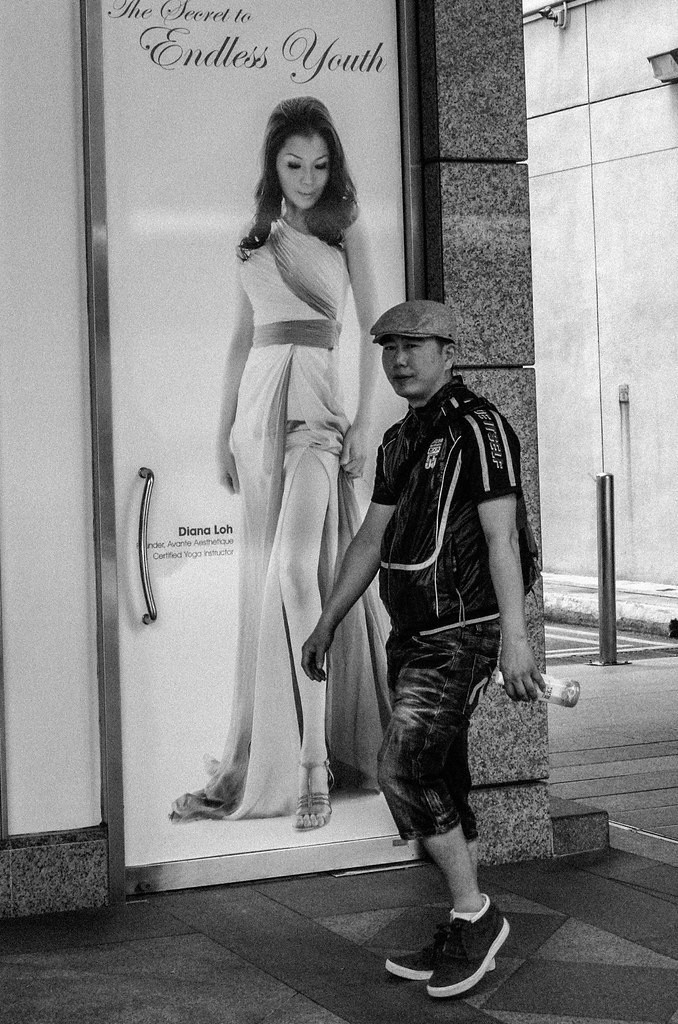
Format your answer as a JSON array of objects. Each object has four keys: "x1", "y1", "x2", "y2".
[{"x1": 370, "y1": 300, "x2": 457, "y2": 344}]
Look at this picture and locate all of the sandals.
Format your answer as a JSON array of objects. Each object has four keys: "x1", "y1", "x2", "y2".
[{"x1": 290, "y1": 758, "x2": 332, "y2": 833}]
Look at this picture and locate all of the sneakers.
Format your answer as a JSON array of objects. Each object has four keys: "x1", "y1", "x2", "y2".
[
  {"x1": 426, "y1": 892, "x2": 510, "y2": 998},
  {"x1": 385, "y1": 923, "x2": 497, "y2": 981}
]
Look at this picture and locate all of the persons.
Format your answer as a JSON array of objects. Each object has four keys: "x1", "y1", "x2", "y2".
[
  {"x1": 301, "y1": 300, "x2": 549, "y2": 999},
  {"x1": 169, "y1": 95, "x2": 394, "y2": 832}
]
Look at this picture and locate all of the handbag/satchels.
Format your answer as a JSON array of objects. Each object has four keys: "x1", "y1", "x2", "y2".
[{"x1": 392, "y1": 395, "x2": 541, "y2": 596}]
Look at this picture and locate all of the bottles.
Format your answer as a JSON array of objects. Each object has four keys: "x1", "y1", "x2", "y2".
[{"x1": 494, "y1": 670, "x2": 579, "y2": 708}]
